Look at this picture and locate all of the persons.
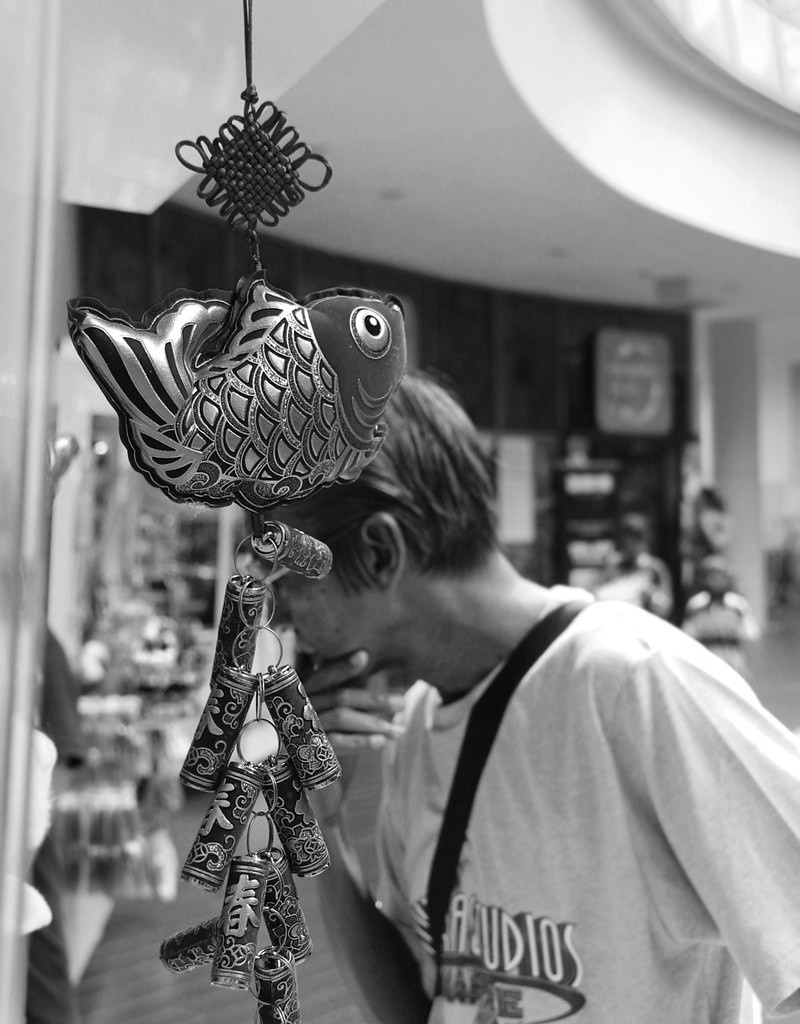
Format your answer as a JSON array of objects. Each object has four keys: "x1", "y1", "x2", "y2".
[
  {"x1": 684, "y1": 560, "x2": 759, "y2": 668},
  {"x1": 260, "y1": 374, "x2": 800, "y2": 1024}
]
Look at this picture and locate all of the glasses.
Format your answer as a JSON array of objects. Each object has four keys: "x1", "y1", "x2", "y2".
[{"x1": 248, "y1": 522, "x2": 359, "y2": 607}]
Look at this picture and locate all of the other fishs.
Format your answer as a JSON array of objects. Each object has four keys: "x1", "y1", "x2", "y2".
[{"x1": 67, "y1": 282, "x2": 407, "y2": 512}]
